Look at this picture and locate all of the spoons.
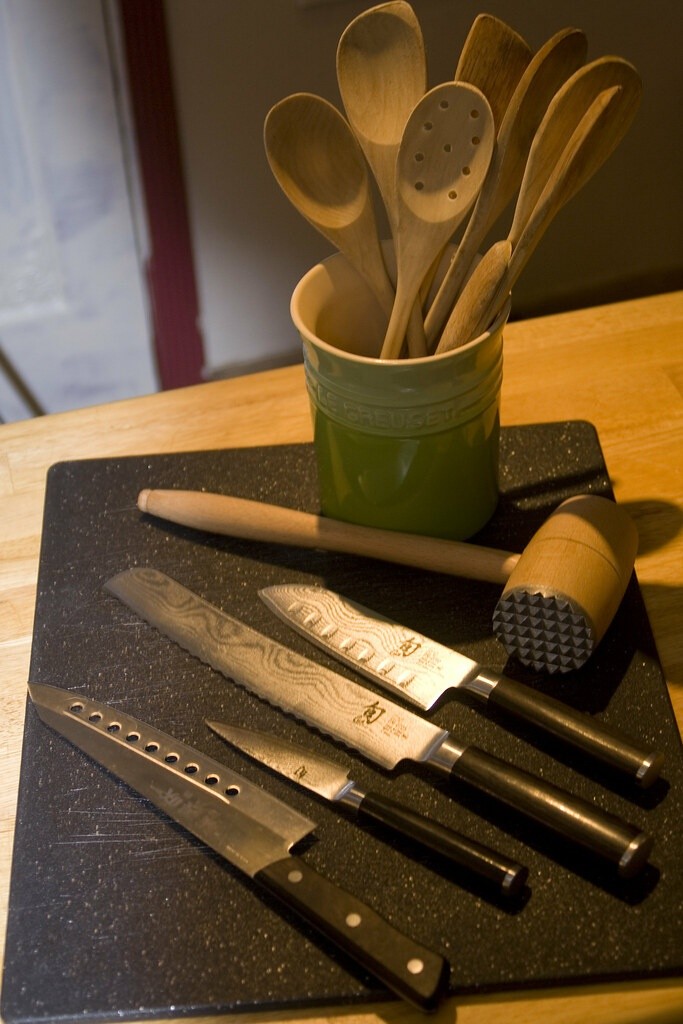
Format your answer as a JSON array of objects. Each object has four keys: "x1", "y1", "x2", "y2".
[
  {"x1": 264, "y1": 92, "x2": 395, "y2": 317},
  {"x1": 335, "y1": 2, "x2": 427, "y2": 240},
  {"x1": 507, "y1": 56, "x2": 643, "y2": 256}
]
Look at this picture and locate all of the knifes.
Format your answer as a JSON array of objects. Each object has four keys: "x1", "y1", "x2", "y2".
[
  {"x1": 204, "y1": 718, "x2": 528, "y2": 907},
  {"x1": 28, "y1": 682, "x2": 450, "y2": 1016},
  {"x1": 103, "y1": 567, "x2": 653, "y2": 871},
  {"x1": 257, "y1": 583, "x2": 665, "y2": 782}
]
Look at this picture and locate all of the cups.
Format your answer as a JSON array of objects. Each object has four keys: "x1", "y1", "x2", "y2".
[{"x1": 289, "y1": 240, "x2": 511, "y2": 546}]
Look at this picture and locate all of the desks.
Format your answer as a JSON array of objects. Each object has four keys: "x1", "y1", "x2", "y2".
[{"x1": 0, "y1": 289, "x2": 683, "y2": 1024}]
